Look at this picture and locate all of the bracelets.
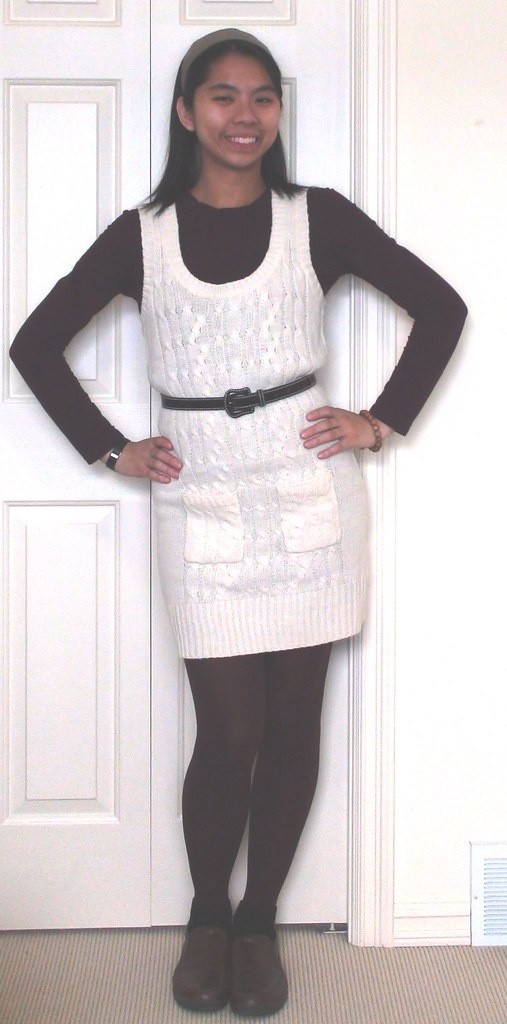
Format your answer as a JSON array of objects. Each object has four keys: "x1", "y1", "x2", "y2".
[
  {"x1": 359, "y1": 410, "x2": 382, "y2": 452},
  {"x1": 105, "y1": 437, "x2": 131, "y2": 471}
]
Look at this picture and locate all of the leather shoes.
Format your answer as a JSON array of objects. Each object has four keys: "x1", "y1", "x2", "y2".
[{"x1": 172, "y1": 896, "x2": 287, "y2": 1017}]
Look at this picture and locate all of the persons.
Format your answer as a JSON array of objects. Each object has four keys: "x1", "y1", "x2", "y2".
[{"x1": 7, "y1": 27, "x2": 468, "y2": 1018}]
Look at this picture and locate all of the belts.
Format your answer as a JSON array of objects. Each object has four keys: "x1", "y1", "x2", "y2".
[{"x1": 161, "y1": 373, "x2": 317, "y2": 418}]
